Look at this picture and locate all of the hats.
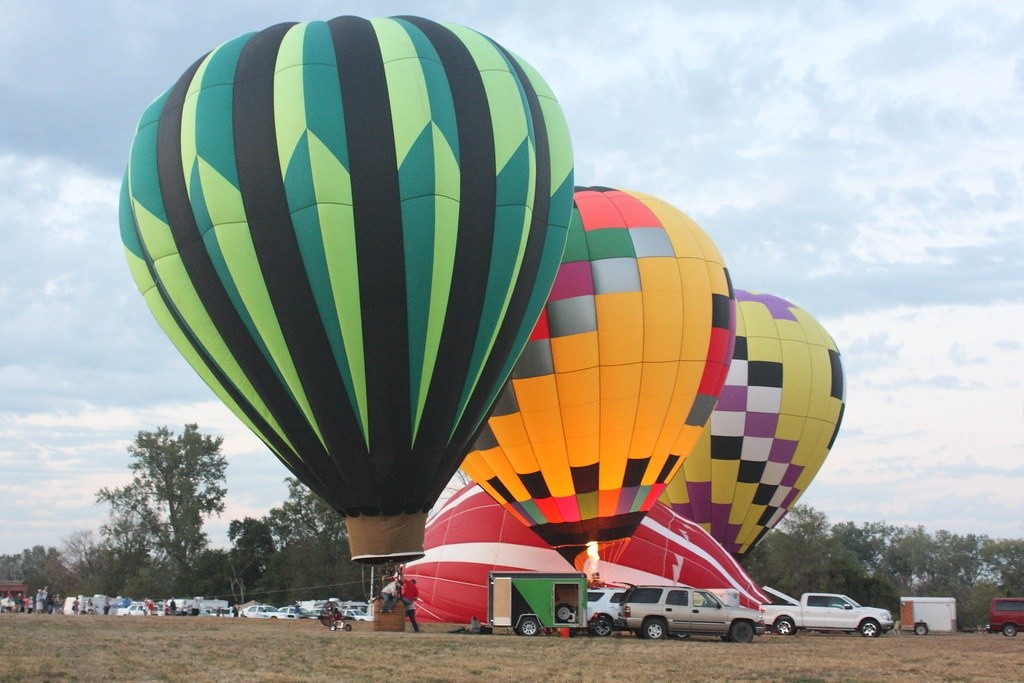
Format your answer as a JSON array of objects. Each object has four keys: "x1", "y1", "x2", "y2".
[
  {"x1": 398, "y1": 579, "x2": 404, "y2": 586},
  {"x1": 77, "y1": 597, "x2": 79, "y2": 599},
  {"x1": 38, "y1": 589, "x2": 42, "y2": 591}
]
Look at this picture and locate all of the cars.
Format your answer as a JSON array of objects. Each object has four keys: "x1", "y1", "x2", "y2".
[{"x1": 117, "y1": 598, "x2": 374, "y2": 622}]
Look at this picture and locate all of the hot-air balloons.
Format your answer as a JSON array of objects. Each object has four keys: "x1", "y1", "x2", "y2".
[
  {"x1": 397, "y1": 479, "x2": 766, "y2": 630},
  {"x1": 458, "y1": 184, "x2": 736, "y2": 586},
  {"x1": 660, "y1": 291, "x2": 849, "y2": 561},
  {"x1": 115, "y1": 14, "x2": 574, "y2": 633}
]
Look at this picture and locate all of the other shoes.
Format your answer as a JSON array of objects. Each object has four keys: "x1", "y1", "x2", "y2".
[
  {"x1": 380, "y1": 609, "x2": 384, "y2": 613},
  {"x1": 387, "y1": 608, "x2": 393, "y2": 612}
]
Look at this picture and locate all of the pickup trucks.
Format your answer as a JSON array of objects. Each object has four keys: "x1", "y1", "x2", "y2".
[{"x1": 760, "y1": 583, "x2": 894, "y2": 638}]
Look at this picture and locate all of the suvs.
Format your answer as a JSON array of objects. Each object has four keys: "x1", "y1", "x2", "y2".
[
  {"x1": 586, "y1": 587, "x2": 691, "y2": 639},
  {"x1": 616, "y1": 586, "x2": 766, "y2": 643}
]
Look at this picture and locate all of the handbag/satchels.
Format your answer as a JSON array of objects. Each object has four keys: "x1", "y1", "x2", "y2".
[{"x1": 72, "y1": 606, "x2": 75, "y2": 610}]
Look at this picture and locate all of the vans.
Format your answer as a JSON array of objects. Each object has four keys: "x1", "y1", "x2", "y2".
[{"x1": 990, "y1": 596, "x2": 1024, "y2": 636}]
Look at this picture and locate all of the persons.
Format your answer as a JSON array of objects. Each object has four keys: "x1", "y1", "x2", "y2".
[
  {"x1": 0, "y1": 585, "x2": 64, "y2": 614},
  {"x1": 72, "y1": 596, "x2": 80, "y2": 614},
  {"x1": 163, "y1": 597, "x2": 177, "y2": 616},
  {"x1": 104, "y1": 595, "x2": 111, "y2": 615},
  {"x1": 182, "y1": 600, "x2": 188, "y2": 616},
  {"x1": 399, "y1": 579, "x2": 420, "y2": 632},
  {"x1": 81, "y1": 599, "x2": 97, "y2": 615},
  {"x1": 192, "y1": 598, "x2": 200, "y2": 616},
  {"x1": 143, "y1": 597, "x2": 154, "y2": 615},
  {"x1": 379, "y1": 579, "x2": 404, "y2": 613}
]
